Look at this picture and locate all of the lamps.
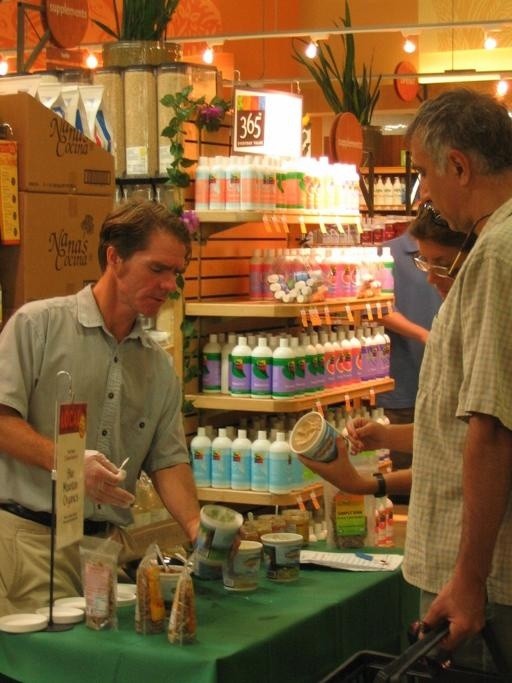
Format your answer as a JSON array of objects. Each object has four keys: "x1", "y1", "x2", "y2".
[{"x1": 0, "y1": 15, "x2": 512, "y2": 77}]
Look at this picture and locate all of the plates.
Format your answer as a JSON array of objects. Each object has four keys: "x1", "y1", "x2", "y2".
[{"x1": 1, "y1": 581, "x2": 139, "y2": 633}]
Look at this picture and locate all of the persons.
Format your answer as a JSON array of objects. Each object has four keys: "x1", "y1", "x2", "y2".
[
  {"x1": 0, "y1": 197, "x2": 242, "y2": 619},
  {"x1": 377, "y1": 233, "x2": 442, "y2": 424},
  {"x1": 295, "y1": 198, "x2": 480, "y2": 498},
  {"x1": 394, "y1": 87, "x2": 512, "y2": 683}
]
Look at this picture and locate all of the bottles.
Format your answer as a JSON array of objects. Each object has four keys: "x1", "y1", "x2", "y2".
[
  {"x1": 363, "y1": 173, "x2": 407, "y2": 206},
  {"x1": 202, "y1": 321, "x2": 392, "y2": 398},
  {"x1": 190, "y1": 405, "x2": 393, "y2": 493},
  {"x1": 247, "y1": 244, "x2": 396, "y2": 300},
  {"x1": 312, "y1": 494, "x2": 395, "y2": 546},
  {"x1": 195, "y1": 153, "x2": 362, "y2": 214}
]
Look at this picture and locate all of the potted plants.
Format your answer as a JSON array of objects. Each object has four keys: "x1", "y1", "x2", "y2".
[{"x1": 85, "y1": 0, "x2": 187, "y2": 66}]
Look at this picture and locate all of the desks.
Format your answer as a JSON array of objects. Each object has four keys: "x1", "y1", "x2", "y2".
[{"x1": 0, "y1": 542, "x2": 427, "y2": 681}]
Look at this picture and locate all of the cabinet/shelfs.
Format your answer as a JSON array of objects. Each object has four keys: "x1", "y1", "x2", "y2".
[
  {"x1": 172, "y1": 203, "x2": 396, "y2": 540},
  {"x1": 4, "y1": 62, "x2": 220, "y2": 211},
  {"x1": 358, "y1": 164, "x2": 433, "y2": 246}
]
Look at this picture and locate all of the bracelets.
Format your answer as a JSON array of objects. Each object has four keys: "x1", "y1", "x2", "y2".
[{"x1": 373, "y1": 473, "x2": 387, "y2": 497}]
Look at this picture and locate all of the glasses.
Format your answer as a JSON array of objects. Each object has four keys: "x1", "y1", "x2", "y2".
[
  {"x1": 413, "y1": 254, "x2": 450, "y2": 278},
  {"x1": 448, "y1": 212, "x2": 491, "y2": 280}
]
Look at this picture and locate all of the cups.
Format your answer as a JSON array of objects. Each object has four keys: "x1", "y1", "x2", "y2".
[{"x1": 158, "y1": 504, "x2": 313, "y2": 604}]
